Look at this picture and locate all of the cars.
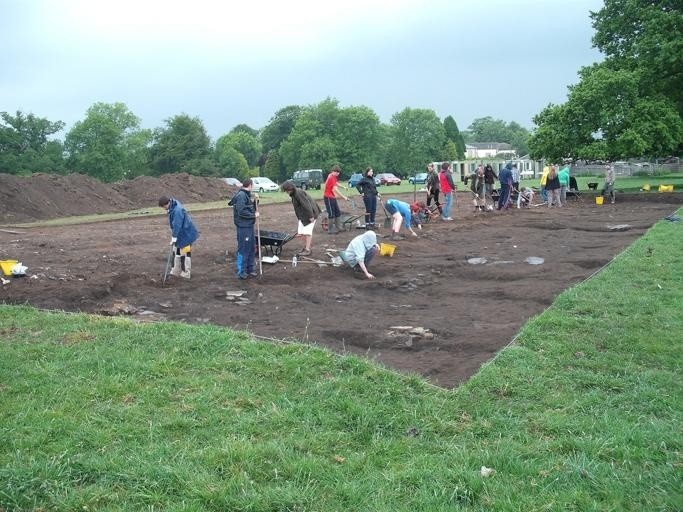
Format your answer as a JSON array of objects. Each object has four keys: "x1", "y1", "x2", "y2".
[
  {"x1": 374, "y1": 172, "x2": 401, "y2": 187},
  {"x1": 247, "y1": 176, "x2": 280, "y2": 194},
  {"x1": 220, "y1": 177, "x2": 244, "y2": 189},
  {"x1": 347, "y1": 173, "x2": 382, "y2": 189},
  {"x1": 408, "y1": 172, "x2": 429, "y2": 184}
]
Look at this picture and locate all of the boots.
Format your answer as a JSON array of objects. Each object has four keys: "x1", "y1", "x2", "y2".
[
  {"x1": 327, "y1": 218, "x2": 338, "y2": 234},
  {"x1": 393, "y1": 233, "x2": 403, "y2": 241},
  {"x1": 334, "y1": 217, "x2": 344, "y2": 231}
]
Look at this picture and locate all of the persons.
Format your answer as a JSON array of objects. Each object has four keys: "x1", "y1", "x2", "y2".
[
  {"x1": 385, "y1": 199, "x2": 422, "y2": 242},
  {"x1": 474, "y1": 164, "x2": 483, "y2": 173},
  {"x1": 464, "y1": 162, "x2": 534, "y2": 213},
  {"x1": 423, "y1": 162, "x2": 441, "y2": 222},
  {"x1": 344, "y1": 230, "x2": 380, "y2": 280},
  {"x1": 355, "y1": 166, "x2": 383, "y2": 230},
  {"x1": 282, "y1": 180, "x2": 322, "y2": 257},
  {"x1": 540, "y1": 165, "x2": 570, "y2": 208},
  {"x1": 158, "y1": 196, "x2": 199, "y2": 280},
  {"x1": 324, "y1": 164, "x2": 349, "y2": 234},
  {"x1": 438, "y1": 162, "x2": 457, "y2": 221},
  {"x1": 600, "y1": 164, "x2": 616, "y2": 204},
  {"x1": 227, "y1": 178, "x2": 260, "y2": 279},
  {"x1": 417, "y1": 202, "x2": 434, "y2": 224}
]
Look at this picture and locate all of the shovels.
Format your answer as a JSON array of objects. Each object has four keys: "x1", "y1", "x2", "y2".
[
  {"x1": 463, "y1": 186, "x2": 503, "y2": 217},
  {"x1": 380, "y1": 199, "x2": 391, "y2": 228}
]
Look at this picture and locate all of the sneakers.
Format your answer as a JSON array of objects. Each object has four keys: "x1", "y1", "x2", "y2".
[
  {"x1": 250, "y1": 271, "x2": 257, "y2": 277},
  {"x1": 241, "y1": 274, "x2": 252, "y2": 280},
  {"x1": 299, "y1": 248, "x2": 311, "y2": 256}
]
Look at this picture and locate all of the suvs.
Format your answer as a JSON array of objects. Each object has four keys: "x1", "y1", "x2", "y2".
[{"x1": 288, "y1": 166, "x2": 323, "y2": 192}]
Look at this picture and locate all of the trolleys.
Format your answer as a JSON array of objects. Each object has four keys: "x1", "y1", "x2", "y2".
[
  {"x1": 321, "y1": 208, "x2": 370, "y2": 233},
  {"x1": 565, "y1": 190, "x2": 584, "y2": 203},
  {"x1": 585, "y1": 182, "x2": 598, "y2": 191},
  {"x1": 491, "y1": 186, "x2": 515, "y2": 207},
  {"x1": 416, "y1": 200, "x2": 447, "y2": 225},
  {"x1": 252, "y1": 230, "x2": 297, "y2": 260}
]
